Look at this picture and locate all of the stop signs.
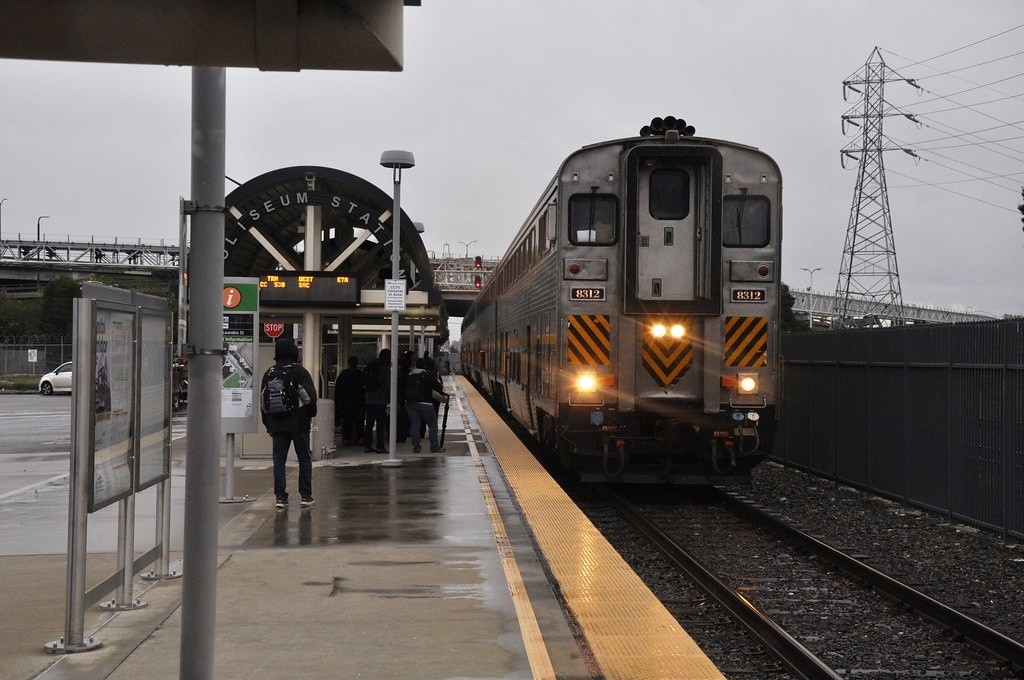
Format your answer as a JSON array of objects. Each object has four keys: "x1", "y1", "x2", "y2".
[{"x1": 264, "y1": 322, "x2": 284, "y2": 337}]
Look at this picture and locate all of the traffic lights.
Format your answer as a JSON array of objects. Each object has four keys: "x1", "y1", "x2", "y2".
[
  {"x1": 475, "y1": 276, "x2": 481, "y2": 289},
  {"x1": 474, "y1": 255, "x2": 481, "y2": 268}
]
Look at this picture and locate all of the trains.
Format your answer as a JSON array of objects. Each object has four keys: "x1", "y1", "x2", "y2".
[{"x1": 460, "y1": 116, "x2": 783, "y2": 490}]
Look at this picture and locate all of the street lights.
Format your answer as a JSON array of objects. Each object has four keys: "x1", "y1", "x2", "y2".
[
  {"x1": 36, "y1": 215, "x2": 50, "y2": 260},
  {"x1": 0, "y1": 197, "x2": 8, "y2": 241},
  {"x1": 458, "y1": 241, "x2": 477, "y2": 259},
  {"x1": 377, "y1": 150, "x2": 416, "y2": 467},
  {"x1": 800, "y1": 267, "x2": 824, "y2": 330}
]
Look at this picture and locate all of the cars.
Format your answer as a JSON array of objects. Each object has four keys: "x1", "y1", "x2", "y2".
[{"x1": 38, "y1": 361, "x2": 73, "y2": 395}]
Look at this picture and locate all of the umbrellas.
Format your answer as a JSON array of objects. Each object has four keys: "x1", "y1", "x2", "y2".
[{"x1": 440, "y1": 395, "x2": 449, "y2": 448}]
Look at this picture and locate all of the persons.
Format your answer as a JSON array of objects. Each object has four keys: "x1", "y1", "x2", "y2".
[
  {"x1": 334, "y1": 349, "x2": 455, "y2": 453},
  {"x1": 260, "y1": 338, "x2": 317, "y2": 509}
]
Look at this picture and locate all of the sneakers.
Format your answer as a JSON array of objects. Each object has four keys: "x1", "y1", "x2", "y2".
[
  {"x1": 300, "y1": 497, "x2": 315, "y2": 505},
  {"x1": 276, "y1": 498, "x2": 289, "y2": 507}
]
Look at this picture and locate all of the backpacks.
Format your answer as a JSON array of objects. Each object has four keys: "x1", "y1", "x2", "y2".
[{"x1": 260, "y1": 362, "x2": 301, "y2": 415}]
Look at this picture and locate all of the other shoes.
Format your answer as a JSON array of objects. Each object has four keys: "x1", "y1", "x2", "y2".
[
  {"x1": 376, "y1": 448, "x2": 389, "y2": 453},
  {"x1": 365, "y1": 448, "x2": 375, "y2": 452},
  {"x1": 431, "y1": 448, "x2": 445, "y2": 452},
  {"x1": 414, "y1": 446, "x2": 421, "y2": 453}
]
